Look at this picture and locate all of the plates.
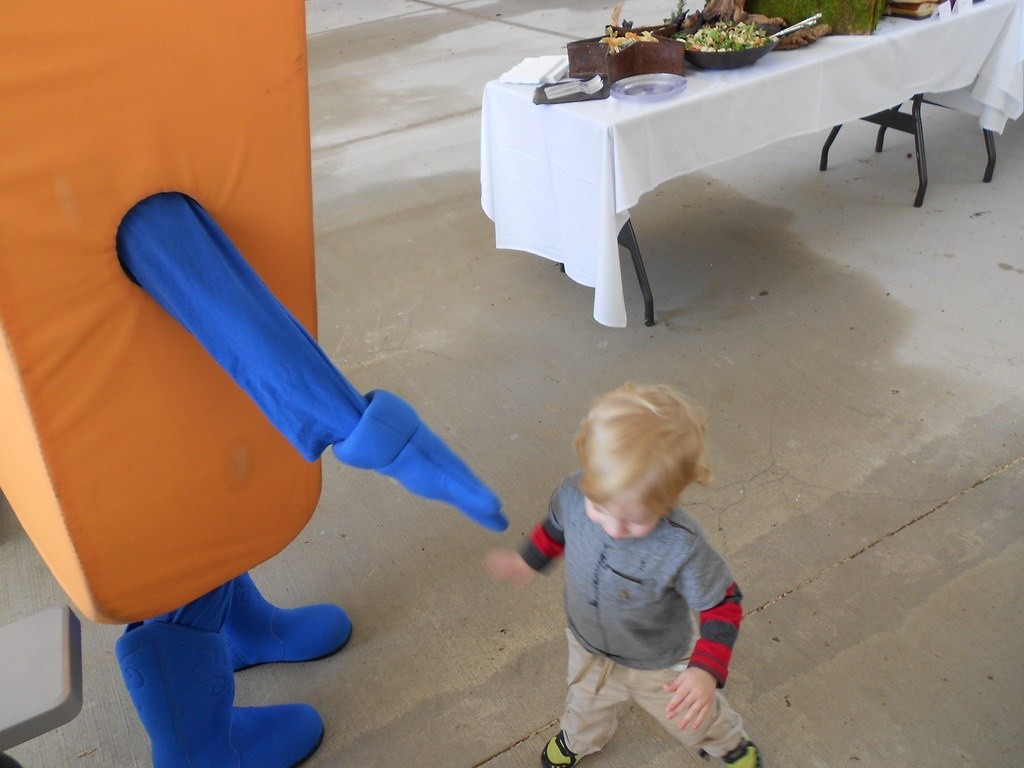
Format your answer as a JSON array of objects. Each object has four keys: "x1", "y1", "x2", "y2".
[{"x1": 609, "y1": 72, "x2": 687, "y2": 104}]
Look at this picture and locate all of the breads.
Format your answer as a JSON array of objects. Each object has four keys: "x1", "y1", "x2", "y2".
[{"x1": 880, "y1": 0, "x2": 939, "y2": 17}]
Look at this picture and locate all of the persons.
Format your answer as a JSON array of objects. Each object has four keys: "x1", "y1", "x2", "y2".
[{"x1": 485, "y1": 385, "x2": 766, "y2": 768}]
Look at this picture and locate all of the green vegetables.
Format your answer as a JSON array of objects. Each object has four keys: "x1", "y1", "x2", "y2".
[{"x1": 673, "y1": 19, "x2": 775, "y2": 50}]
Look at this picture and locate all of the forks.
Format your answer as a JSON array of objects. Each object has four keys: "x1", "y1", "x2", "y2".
[{"x1": 543, "y1": 74, "x2": 603, "y2": 99}]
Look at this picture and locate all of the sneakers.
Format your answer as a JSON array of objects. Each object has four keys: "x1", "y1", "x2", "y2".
[
  {"x1": 700, "y1": 731, "x2": 764, "y2": 768},
  {"x1": 542, "y1": 727, "x2": 607, "y2": 768}
]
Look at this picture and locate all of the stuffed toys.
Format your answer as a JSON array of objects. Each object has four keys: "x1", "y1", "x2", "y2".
[{"x1": 0, "y1": 0, "x2": 510, "y2": 768}]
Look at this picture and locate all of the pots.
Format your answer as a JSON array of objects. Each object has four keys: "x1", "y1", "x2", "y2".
[{"x1": 671, "y1": 22, "x2": 783, "y2": 69}]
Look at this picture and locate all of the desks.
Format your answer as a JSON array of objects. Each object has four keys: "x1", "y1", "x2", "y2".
[{"x1": 474, "y1": 0, "x2": 1024, "y2": 328}]
